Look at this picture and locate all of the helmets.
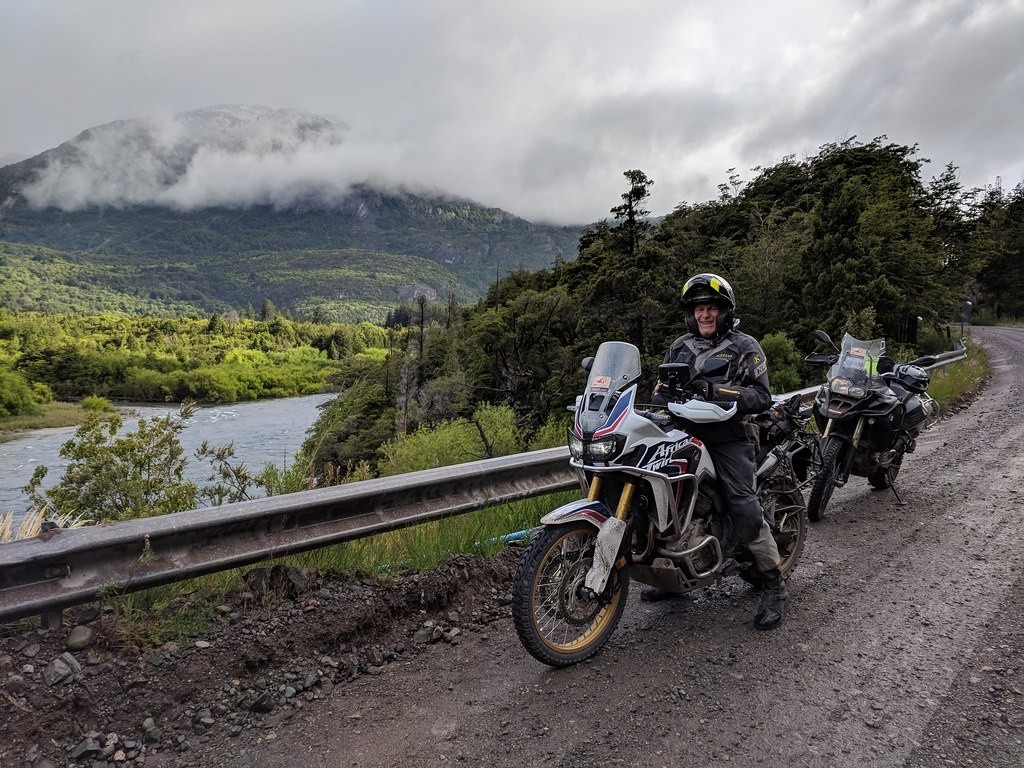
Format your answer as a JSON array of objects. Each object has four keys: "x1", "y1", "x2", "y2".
[{"x1": 679, "y1": 273, "x2": 736, "y2": 338}]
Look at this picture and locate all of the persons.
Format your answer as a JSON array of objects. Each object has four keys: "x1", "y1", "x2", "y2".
[{"x1": 641, "y1": 274, "x2": 789, "y2": 630}]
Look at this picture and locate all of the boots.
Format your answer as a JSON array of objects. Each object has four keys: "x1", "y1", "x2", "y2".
[
  {"x1": 753, "y1": 562, "x2": 790, "y2": 630},
  {"x1": 641, "y1": 586, "x2": 681, "y2": 601}
]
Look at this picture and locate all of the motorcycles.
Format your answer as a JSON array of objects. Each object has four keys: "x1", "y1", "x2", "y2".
[
  {"x1": 514, "y1": 340, "x2": 825, "y2": 668},
  {"x1": 804, "y1": 327, "x2": 941, "y2": 525}
]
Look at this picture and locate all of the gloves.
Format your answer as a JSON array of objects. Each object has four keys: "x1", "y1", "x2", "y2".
[{"x1": 691, "y1": 380, "x2": 707, "y2": 398}]
